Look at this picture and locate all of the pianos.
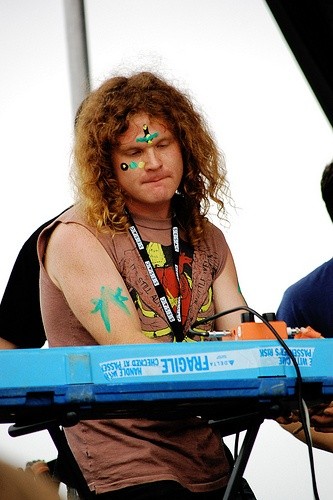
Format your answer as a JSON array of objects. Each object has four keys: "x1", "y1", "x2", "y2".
[{"x1": 1, "y1": 310, "x2": 333, "y2": 500}]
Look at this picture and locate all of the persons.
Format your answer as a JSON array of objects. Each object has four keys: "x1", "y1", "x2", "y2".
[
  {"x1": 37, "y1": 72, "x2": 313, "y2": 500},
  {"x1": 271, "y1": 158, "x2": 333, "y2": 453},
  {"x1": 0, "y1": 203, "x2": 92, "y2": 500}
]
